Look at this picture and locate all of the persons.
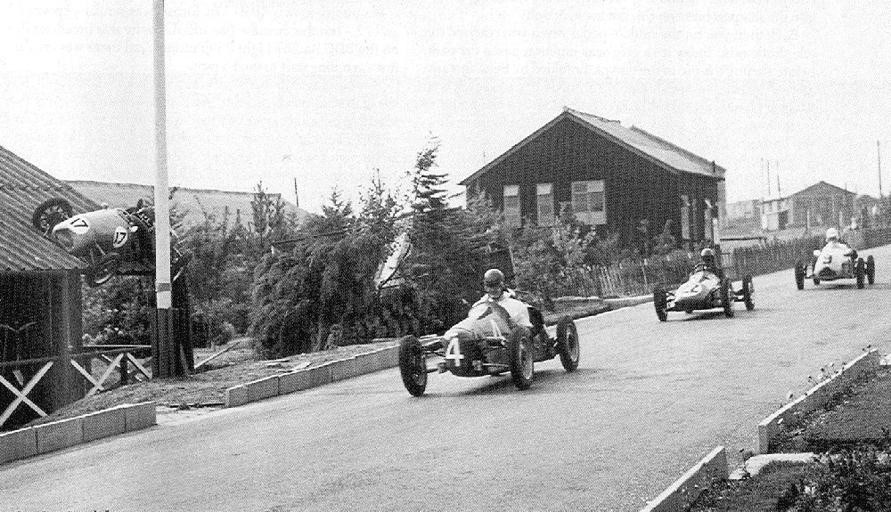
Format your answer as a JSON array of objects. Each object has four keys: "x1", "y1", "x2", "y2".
[
  {"x1": 691, "y1": 247, "x2": 724, "y2": 276},
  {"x1": 824, "y1": 227, "x2": 848, "y2": 254},
  {"x1": 469, "y1": 266, "x2": 534, "y2": 332}
]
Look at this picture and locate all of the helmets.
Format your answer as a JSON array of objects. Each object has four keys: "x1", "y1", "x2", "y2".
[
  {"x1": 482, "y1": 267, "x2": 507, "y2": 288},
  {"x1": 826, "y1": 226, "x2": 839, "y2": 240},
  {"x1": 700, "y1": 247, "x2": 715, "y2": 258}
]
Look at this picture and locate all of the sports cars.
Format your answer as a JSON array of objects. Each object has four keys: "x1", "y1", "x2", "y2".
[
  {"x1": 654, "y1": 269, "x2": 754, "y2": 321},
  {"x1": 397, "y1": 303, "x2": 579, "y2": 397},
  {"x1": 32, "y1": 206, "x2": 191, "y2": 285},
  {"x1": 794, "y1": 248, "x2": 876, "y2": 290}
]
